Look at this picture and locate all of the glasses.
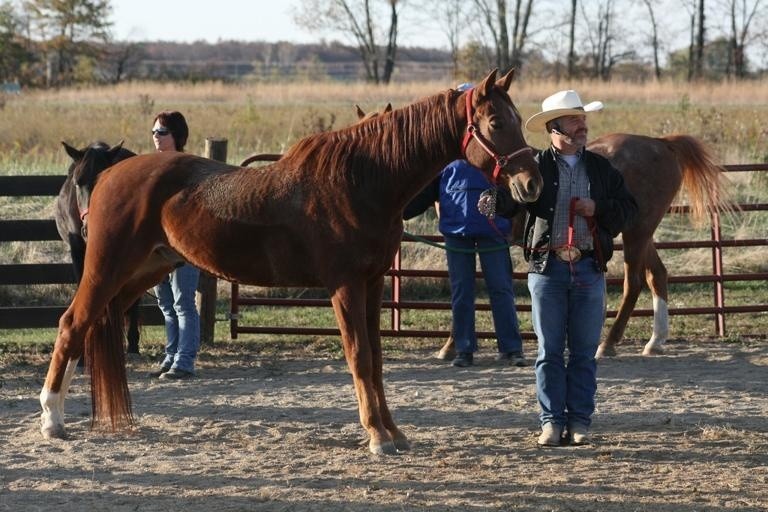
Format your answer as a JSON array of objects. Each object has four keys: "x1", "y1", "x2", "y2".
[{"x1": 151, "y1": 126, "x2": 169, "y2": 136}]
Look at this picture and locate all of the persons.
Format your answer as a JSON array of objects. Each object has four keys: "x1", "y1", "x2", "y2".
[
  {"x1": 477, "y1": 90, "x2": 640, "y2": 447},
  {"x1": 430, "y1": 79, "x2": 526, "y2": 368},
  {"x1": 143, "y1": 112, "x2": 205, "y2": 381}
]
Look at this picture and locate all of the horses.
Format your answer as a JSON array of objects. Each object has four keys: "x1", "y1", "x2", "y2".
[
  {"x1": 356, "y1": 102, "x2": 745, "y2": 360},
  {"x1": 40, "y1": 68, "x2": 543, "y2": 455},
  {"x1": 55, "y1": 141, "x2": 141, "y2": 374}
]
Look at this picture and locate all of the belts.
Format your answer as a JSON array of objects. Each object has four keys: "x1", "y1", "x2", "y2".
[{"x1": 550, "y1": 243, "x2": 597, "y2": 264}]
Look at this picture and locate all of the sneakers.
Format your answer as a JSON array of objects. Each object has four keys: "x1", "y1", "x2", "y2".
[
  {"x1": 148, "y1": 368, "x2": 191, "y2": 381},
  {"x1": 454, "y1": 354, "x2": 473, "y2": 368},
  {"x1": 538, "y1": 421, "x2": 591, "y2": 447},
  {"x1": 498, "y1": 351, "x2": 528, "y2": 366}
]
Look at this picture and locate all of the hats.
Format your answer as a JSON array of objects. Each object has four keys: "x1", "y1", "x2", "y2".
[{"x1": 524, "y1": 90, "x2": 604, "y2": 134}]
